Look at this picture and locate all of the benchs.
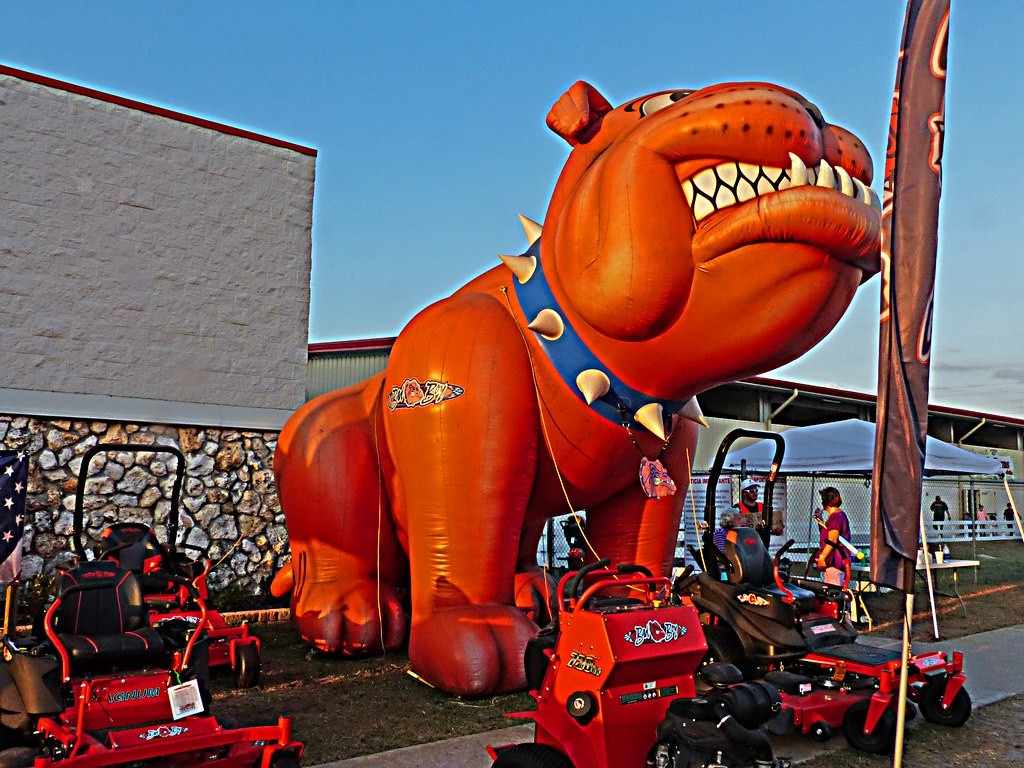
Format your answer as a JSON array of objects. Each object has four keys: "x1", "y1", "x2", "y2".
[{"x1": 791, "y1": 574, "x2": 893, "y2": 625}]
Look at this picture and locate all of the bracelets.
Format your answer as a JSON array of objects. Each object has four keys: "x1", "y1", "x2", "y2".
[{"x1": 819, "y1": 554, "x2": 826, "y2": 558}]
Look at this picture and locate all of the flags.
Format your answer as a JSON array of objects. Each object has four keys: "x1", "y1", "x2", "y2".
[{"x1": 0, "y1": 449, "x2": 29, "y2": 588}]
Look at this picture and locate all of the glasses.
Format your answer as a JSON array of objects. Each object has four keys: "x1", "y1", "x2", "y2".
[{"x1": 744, "y1": 488, "x2": 759, "y2": 493}]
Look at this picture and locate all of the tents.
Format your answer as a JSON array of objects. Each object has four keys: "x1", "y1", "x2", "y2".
[{"x1": 709, "y1": 418, "x2": 1024, "y2": 642}]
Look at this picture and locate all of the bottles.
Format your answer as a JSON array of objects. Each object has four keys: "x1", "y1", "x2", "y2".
[
  {"x1": 861, "y1": 549, "x2": 867, "y2": 566},
  {"x1": 943, "y1": 543, "x2": 950, "y2": 563}
]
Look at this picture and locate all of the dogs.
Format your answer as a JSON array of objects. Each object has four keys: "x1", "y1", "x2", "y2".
[{"x1": 267, "y1": 77, "x2": 884, "y2": 692}]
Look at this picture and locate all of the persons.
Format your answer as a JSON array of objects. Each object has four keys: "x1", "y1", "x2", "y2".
[
  {"x1": 812, "y1": 486, "x2": 852, "y2": 586},
  {"x1": 1003, "y1": 503, "x2": 1022, "y2": 537},
  {"x1": 976, "y1": 503, "x2": 988, "y2": 538},
  {"x1": 930, "y1": 495, "x2": 952, "y2": 538},
  {"x1": 712, "y1": 479, "x2": 784, "y2": 571}
]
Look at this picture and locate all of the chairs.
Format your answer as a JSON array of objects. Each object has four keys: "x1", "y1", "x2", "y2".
[
  {"x1": 57, "y1": 561, "x2": 163, "y2": 673},
  {"x1": 726, "y1": 526, "x2": 816, "y2": 620},
  {"x1": 101, "y1": 524, "x2": 174, "y2": 592}
]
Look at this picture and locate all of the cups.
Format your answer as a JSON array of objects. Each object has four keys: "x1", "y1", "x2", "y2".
[
  {"x1": 916, "y1": 550, "x2": 923, "y2": 565},
  {"x1": 935, "y1": 551, "x2": 944, "y2": 564}
]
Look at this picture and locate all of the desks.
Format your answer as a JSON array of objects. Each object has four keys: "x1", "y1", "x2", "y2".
[{"x1": 850, "y1": 558, "x2": 980, "y2": 618}]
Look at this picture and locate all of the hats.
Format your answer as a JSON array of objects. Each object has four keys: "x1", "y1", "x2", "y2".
[{"x1": 741, "y1": 479, "x2": 761, "y2": 491}]
